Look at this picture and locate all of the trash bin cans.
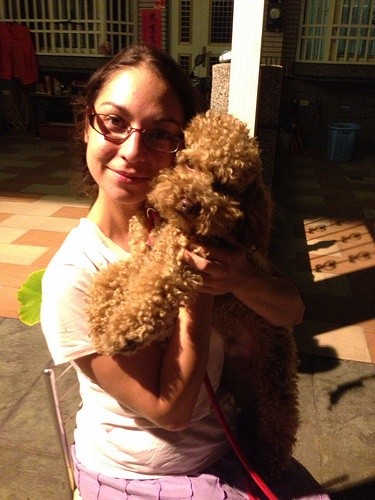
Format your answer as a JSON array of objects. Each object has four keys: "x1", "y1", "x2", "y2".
[{"x1": 324, "y1": 120, "x2": 362, "y2": 163}]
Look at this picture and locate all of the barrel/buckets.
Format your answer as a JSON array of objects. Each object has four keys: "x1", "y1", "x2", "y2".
[{"x1": 326, "y1": 122, "x2": 361, "y2": 162}]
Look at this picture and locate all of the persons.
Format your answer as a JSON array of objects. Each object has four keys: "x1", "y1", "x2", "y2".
[{"x1": 40, "y1": 46, "x2": 331, "y2": 498}]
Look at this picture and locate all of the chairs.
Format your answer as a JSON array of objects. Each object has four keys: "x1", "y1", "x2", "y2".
[{"x1": 43, "y1": 364, "x2": 82, "y2": 500}]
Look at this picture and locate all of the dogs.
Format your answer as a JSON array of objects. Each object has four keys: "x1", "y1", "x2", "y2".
[{"x1": 82, "y1": 107, "x2": 301, "y2": 481}]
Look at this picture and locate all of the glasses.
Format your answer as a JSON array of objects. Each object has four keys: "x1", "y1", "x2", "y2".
[{"x1": 88, "y1": 103, "x2": 185, "y2": 154}]
